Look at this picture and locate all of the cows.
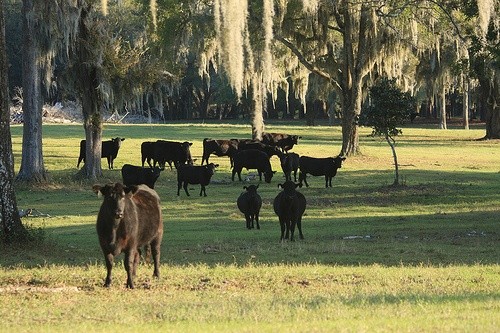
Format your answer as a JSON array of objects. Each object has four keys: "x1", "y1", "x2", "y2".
[
  {"x1": 121, "y1": 163, "x2": 165, "y2": 189},
  {"x1": 299, "y1": 155, "x2": 345, "y2": 188},
  {"x1": 139, "y1": 132, "x2": 300, "y2": 182},
  {"x1": 177, "y1": 162, "x2": 219, "y2": 198},
  {"x1": 77, "y1": 137, "x2": 125, "y2": 170},
  {"x1": 231, "y1": 151, "x2": 277, "y2": 184},
  {"x1": 273, "y1": 180, "x2": 307, "y2": 241},
  {"x1": 237, "y1": 183, "x2": 262, "y2": 229},
  {"x1": 92, "y1": 182, "x2": 163, "y2": 289}
]
